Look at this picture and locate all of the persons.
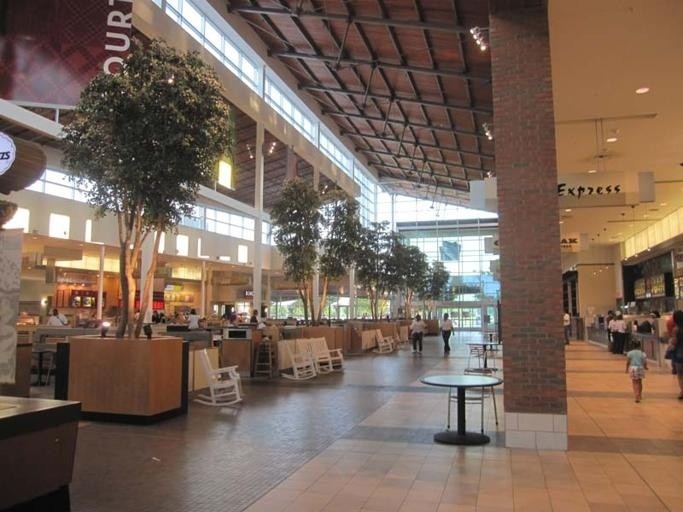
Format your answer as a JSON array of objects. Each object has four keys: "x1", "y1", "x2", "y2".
[
  {"x1": 408, "y1": 314, "x2": 428, "y2": 354},
  {"x1": 666, "y1": 314, "x2": 677, "y2": 374},
  {"x1": 47, "y1": 308, "x2": 68, "y2": 326},
  {"x1": 183, "y1": 308, "x2": 201, "y2": 330},
  {"x1": 669, "y1": 309, "x2": 683, "y2": 399},
  {"x1": 563, "y1": 310, "x2": 572, "y2": 346},
  {"x1": 647, "y1": 310, "x2": 660, "y2": 335},
  {"x1": 239, "y1": 314, "x2": 243, "y2": 323},
  {"x1": 249, "y1": 309, "x2": 259, "y2": 326},
  {"x1": 220, "y1": 314, "x2": 226, "y2": 320},
  {"x1": 134, "y1": 308, "x2": 168, "y2": 324},
  {"x1": 625, "y1": 340, "x2": 649, "y2": 403},
  {"x1": 605, "y1": 307, "x2": 628, "y2": 355},
  {"x1": 439, "y1": 312, "x2": 455, "y2": 355}
]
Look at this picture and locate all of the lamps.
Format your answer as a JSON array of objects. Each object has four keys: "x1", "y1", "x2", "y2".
[
  {"x1": 208, "y1": 148, "x2": 238, "y2": 193},
  {"x1": 470, "y1": 26, "x2": 490, "y2": 52},
  {"x1": 262, "y1": 141, "x2": 277, "y2": 156},
  {"x1": 487, "y1": 171, "x2": 494, "y2": 178},
  {"x1": 483, "y1": 123, "x2": 494, "y2": 142}
]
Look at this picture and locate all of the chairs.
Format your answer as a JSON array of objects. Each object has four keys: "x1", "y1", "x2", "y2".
[
  {"x1": 281, "y1": 342, "x2": 320, "y2": 380},
  {"x1": 193, "y1": 349, "x2": 244, "y2": 407},
  {"x1": 372, "y1": 329, "x2": 397, "y2": 353},
  {"x1": 314, "y1": 339, "x2": 347, "y2": 375}
]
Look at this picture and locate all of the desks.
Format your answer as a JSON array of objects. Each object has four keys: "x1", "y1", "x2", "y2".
[
  {"x1": 465, "y1": 341, "x2": 502, "y2": 370},
  {"x1": 418, "y1": 374, "x2": 503, "y2": 446}
]
